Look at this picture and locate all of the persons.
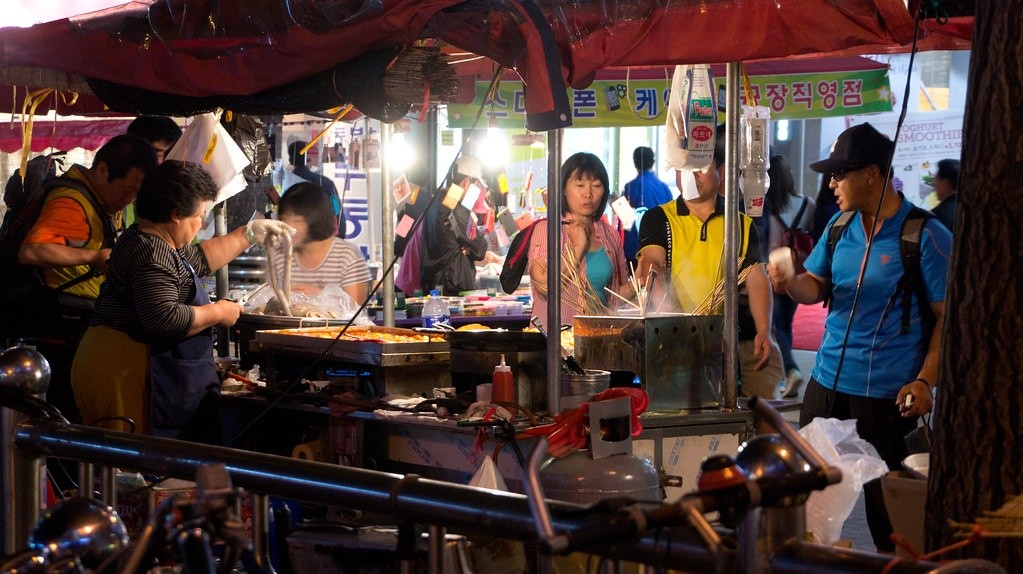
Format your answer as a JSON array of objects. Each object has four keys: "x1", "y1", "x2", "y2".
[{"x1": 0, "y1": 116, "x2": 959, "y2": 551}]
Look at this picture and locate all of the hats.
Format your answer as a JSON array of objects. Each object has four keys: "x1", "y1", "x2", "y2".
[
  {"x1": 809, "y1": 122, "x2": 896, "y2": 176},
  {"x1": 453, "y1": 156, "x2": 488, "y2": 189}
]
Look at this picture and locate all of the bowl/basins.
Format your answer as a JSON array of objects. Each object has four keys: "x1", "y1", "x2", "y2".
[{"x1": 433, "y1": 388, "x2": 456, "y2": 399}]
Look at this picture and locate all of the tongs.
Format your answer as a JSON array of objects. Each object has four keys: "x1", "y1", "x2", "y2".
[{"x1": 531, "y1": 315, "x2": 587, "y2": 376}]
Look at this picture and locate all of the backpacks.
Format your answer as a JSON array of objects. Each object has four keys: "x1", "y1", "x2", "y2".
[
  {"x1": 0, "y1": 155, "x2": 116, "y2": 338},
  {"x1": 772, "y1": 195, "x2": 816, "y2": 276}
]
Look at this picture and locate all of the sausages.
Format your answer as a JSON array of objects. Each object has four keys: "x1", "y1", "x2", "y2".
[{"x1": 264, "y1": 227, "x2": 293, "y2": 318}]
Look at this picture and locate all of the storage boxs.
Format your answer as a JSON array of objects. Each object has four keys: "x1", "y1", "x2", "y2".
[{"x1": 95, "y1": 472, "x2": 254, "y2": 571}]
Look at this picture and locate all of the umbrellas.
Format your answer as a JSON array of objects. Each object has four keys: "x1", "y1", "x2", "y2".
[
  {"x1": 385, "y1": 0, "x2": 983, "y2": 418},
  {"x1": 1, "y1": 0, "x2": 673, "y2": 419}
]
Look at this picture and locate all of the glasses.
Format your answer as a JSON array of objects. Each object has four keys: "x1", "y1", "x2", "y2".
[{"x1": 830, "y1": 164, "x2": 867, "y2": 181}]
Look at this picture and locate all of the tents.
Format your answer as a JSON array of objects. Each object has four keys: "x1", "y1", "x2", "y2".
[{"x1": 1, "y1": 114, "x2": 188, "y2": 224}]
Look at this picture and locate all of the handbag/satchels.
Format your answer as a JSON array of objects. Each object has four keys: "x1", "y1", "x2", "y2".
[{"x1": 664, "y1": 62, "x2": 717, "y2": 175}]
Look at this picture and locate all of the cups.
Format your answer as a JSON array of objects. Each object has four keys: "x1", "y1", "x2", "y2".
[{"x1": 768, "y1": 247, "x2": 795, "y2": 283}]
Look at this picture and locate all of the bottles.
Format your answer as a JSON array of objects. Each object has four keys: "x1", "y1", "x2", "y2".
[
  {"x1": 336, "y1": 143, "x2": 346, "y2": 169},
  {"x1": 421, "y1": 289, "x2": 451, "y2": 329},
  {"x1": 353, "y1": 138, "x2": 362, "y2": 170},
  {"x1": 322, "y1": 144, "x2": 331, "y2": 163},
  {"x1": 491, "y1": 354, "x2": 515, "y2": 418}
]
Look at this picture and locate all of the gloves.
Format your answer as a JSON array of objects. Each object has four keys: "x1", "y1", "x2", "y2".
[{"x1": 525, "y1": 387, "x2": 648, "y2": 460}]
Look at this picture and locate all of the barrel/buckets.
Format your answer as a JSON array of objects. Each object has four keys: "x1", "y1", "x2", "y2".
[{"x1": 562, "y1": 369, "x2": 611, "y2": 396}]
